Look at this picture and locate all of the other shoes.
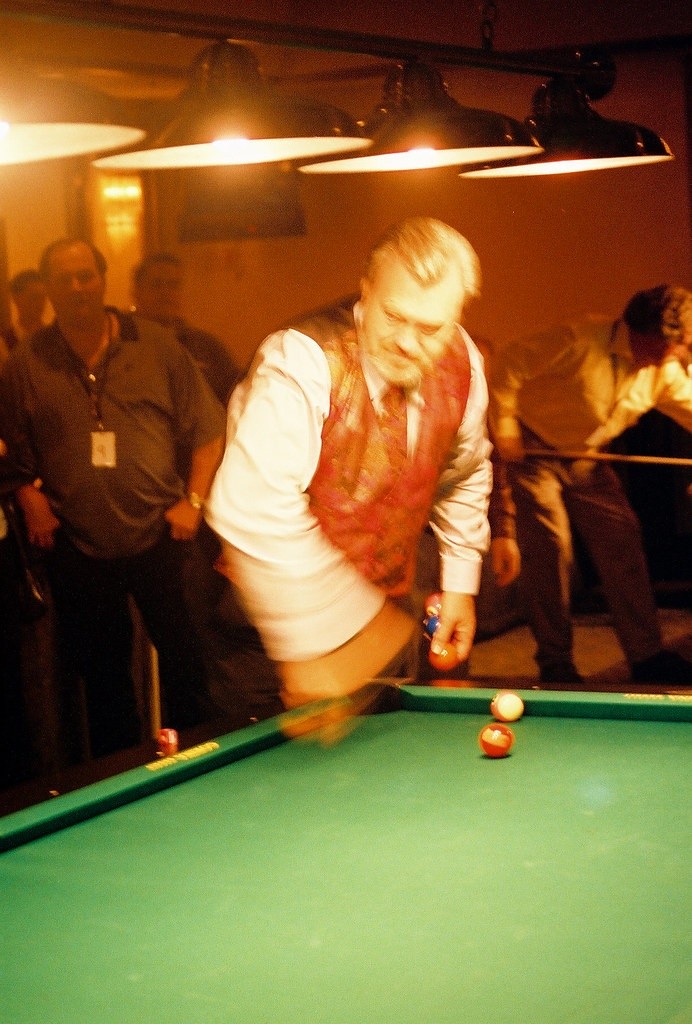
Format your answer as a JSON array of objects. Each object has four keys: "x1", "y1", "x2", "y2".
[
  {"x1": 541, "y1": 669, "x2": 582, "y2": 684},
  {"x1": 632, "y1": 649, "x2": 692, "y2": 685}
]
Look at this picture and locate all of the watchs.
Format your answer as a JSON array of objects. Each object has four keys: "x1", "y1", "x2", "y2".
[{"x1": 185, "y1": 489, "x2": 203, "y2": 510}]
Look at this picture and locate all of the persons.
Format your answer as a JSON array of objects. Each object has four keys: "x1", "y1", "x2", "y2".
[
  {"x1": 202, "y1": 214, "x2": 495, "y2": 734},
  {"x1": 487, "y1": 282, "x2": 692, "y2": 685},
  {"x1": 0, "y1": 238, "x2": 234, "y2": 786}
]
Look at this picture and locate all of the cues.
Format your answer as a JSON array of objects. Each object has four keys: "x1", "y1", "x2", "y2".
[{"x1": 523, "y1": 446, "x2": 692, "y2": 467}]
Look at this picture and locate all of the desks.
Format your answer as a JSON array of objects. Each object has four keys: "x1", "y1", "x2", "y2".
[{"x1": 0, "y1": 674, "x2": 692, "y2": 1024}]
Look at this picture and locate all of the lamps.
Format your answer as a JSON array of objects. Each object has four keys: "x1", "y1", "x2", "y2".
[
  {"x1": 87, "y1": 34, "x2": 374, "y2": 172},
  {"x1": 0, "y1": 23, "x2": 155, "y2": 170},
  {"x1": 291, "y1": 60, "x2": 546, "y2": 174},
  {"x1": 456, "y1": 78, "x2": 675, "y2": 180}
]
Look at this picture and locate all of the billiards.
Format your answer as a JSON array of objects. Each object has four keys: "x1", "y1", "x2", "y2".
[
  {"x1": 490, "y1": 689, "x2": 525, "y2": 723},
  {"x1": 425, "y1": 592, "x2": 459, "y2": 672},
  {"x1": 477, "y1": 722, "x2": 514, "y2": 759}
]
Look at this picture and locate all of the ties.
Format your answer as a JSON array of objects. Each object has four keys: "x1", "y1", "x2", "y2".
[{"x1": 378, "y1": 384, "x2": 406, "y2": 458}]
